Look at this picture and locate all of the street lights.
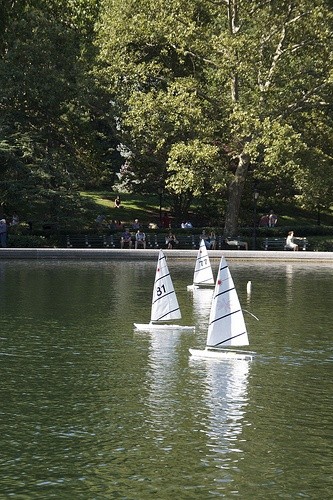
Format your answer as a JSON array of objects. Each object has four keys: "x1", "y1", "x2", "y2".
[
  {"x1": 252, "y1": 189, "x2": 260, "y2": 250},
  {"x1": 157, "y1": 186, "x2": 164, "y2": 222}
]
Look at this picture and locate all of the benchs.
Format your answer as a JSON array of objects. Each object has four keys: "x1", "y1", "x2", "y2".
[
  {"x1": 66, "y1": 234, "x2": 245, "y2": 251},
  {"x1": 260, "y1": 237, "x2": 309, "y2": 253}
]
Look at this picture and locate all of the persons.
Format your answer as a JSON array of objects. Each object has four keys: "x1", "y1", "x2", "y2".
[
  {"x1": 120, "y1": 228, "x2": 157, "y2": 249},
  {"x1": 12, "y1": 212, "x2": 19, "y2": 225},
  {"x1": 166, "y1": 232, "x2": 176, "y2": 249},
  {"x1": 114, "y1": 196, "x2": 120, "y2": 208},
  {"x1": 94, "y1": 211, "x2": 109, "y2": 227},
  {"x1": 107, "y1": 218, "x2": 142, "y2": 228},
  {"x1": 225, "y1": 238, "x2": 248, "y2": 250},
  {"x1": 162, "y1": 213, "x2": 170, "y2": 226},
  {"x1": 269, "y1": 210, "x2": 278, "y2": 224},
  {"x1": 180, "y1": 220, "x2": 192, "y2": 228},
  {"x1": 0, "y1": 219, "x2": 8, "y2": 248},
  {"x1": 199, "y1": 229, "x2": 217, "y2": 250},
  {"x1": 286, "y1": 231, "x2": 298, "y2": 251},
  {"x1": 258, "y1": 213, "x2": 269, "y2": 227}
]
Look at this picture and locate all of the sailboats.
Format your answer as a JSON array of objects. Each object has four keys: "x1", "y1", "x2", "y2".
[
  {"x1": 187, "y1": 238, "x2": 216, "y2": 289},
  {"x1": 133, "y1": 248, "x2": 196, "y2": 330},
  {"x1": 188, "y1": 256, "x2": 258, "y2": 360}
]
